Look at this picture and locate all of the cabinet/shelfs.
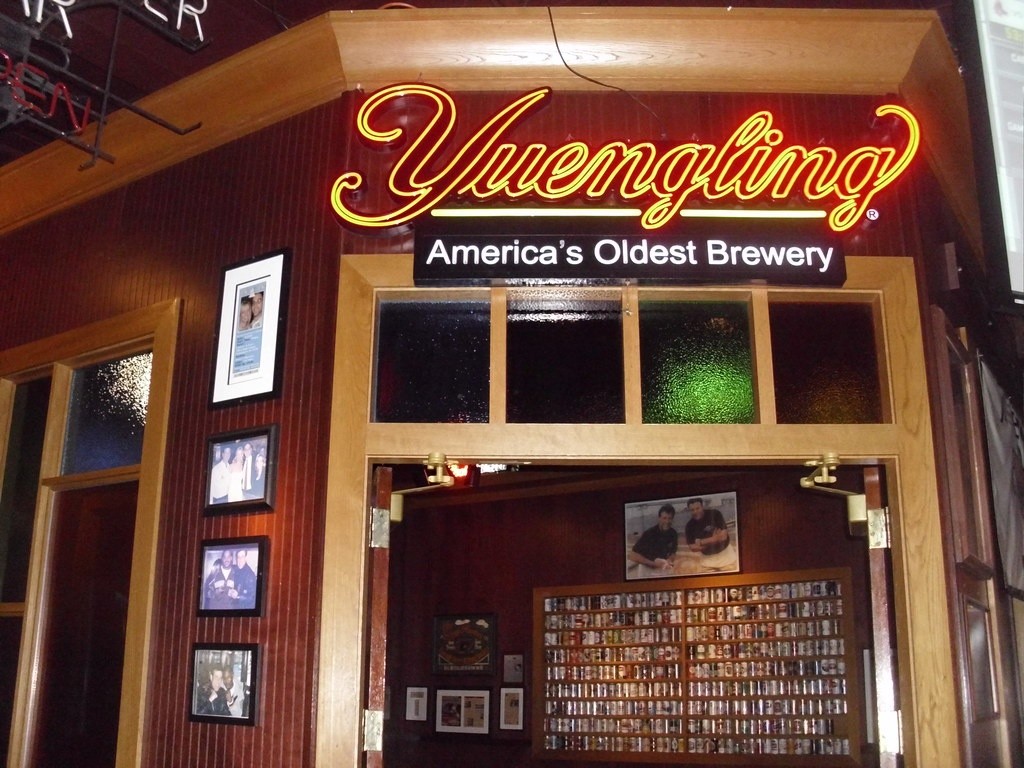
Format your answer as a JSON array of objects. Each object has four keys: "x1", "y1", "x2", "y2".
[{"x1": 532, "y1": 566, "x2": 864, "y2": 768}]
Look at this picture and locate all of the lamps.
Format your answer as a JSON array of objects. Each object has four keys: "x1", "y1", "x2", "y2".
[{"x1": 465, "y1": 464, "x2": 482, "y2": 488}]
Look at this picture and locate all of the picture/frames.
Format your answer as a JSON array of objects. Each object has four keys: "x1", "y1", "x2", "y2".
[
  {"x1": 404, "y1": 684, "x2": 429, "y2": 723},
  {"x1": 499, "y1": 687, "x2": 524, "y2": 730},
  {"x1": 198, "y1": 424, "x2": 278, "y2": 515},
  {"x1": 187, "y1": 641, "x2": 259, "y2": 727},
  {"x1": 205, "y1": 248, "x2": 285, "y2": 411},
  {"x1": 432, "y1": 686, "x2": 493, "y2": 735},
  {"x1": 431, "y1": 613, "x2": 498, "y2": 676},
  {"x1": 192, "y1": 535, "x2": 266, "y2": 616}
]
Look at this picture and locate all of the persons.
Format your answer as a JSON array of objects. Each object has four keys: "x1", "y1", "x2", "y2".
[
  {"x1": 210, "y1": 444, "x2": 267, "y2": 504},
  {"x1": 629, "y1": 505, "x2": 678, "y2": 569},
  {"x1": 203, "y1": 548, "x2": 256, "y2": 609},
  {"x1": 686, "y1": 498, "x2": 738, "y2": 573},
  {"x1": 238, "y1": 292, "x2": 263, "y2": 329},
  {"x1": 195, "y1": 650, "x2": 244, "y2": 717}
]
